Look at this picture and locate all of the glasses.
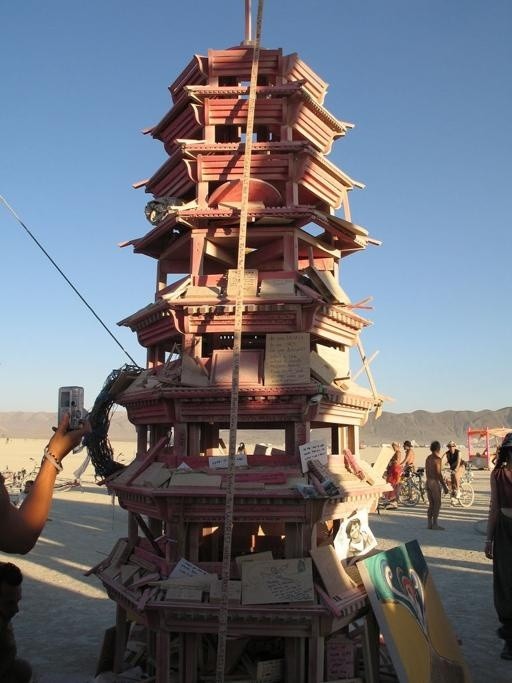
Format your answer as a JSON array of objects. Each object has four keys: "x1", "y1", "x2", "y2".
[{"x1": 448, "y1": 446, "x2": 455, "y2": 448}]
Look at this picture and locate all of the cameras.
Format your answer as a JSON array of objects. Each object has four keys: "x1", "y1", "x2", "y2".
[{"x1": 58, "y1": 386, "x2": 85, "y2": 429}]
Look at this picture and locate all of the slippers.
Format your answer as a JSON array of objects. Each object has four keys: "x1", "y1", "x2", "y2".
[{"x1": 432, "y1": 526, "x2": 445, "y2": 530}]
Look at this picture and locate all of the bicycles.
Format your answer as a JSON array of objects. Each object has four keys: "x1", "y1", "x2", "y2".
[
  {"x1": 21, "y1": 457, "x2": 41, "y2": 494},
  {"x1": 375, "y1": 465, "x2": 474, "y2": 508}
]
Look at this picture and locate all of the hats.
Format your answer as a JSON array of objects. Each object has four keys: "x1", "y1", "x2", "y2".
[
  {"x1": 447, "y1": 440, "x2": 457, "y2": 447},
  {"x1": 404, "y1": 441, "x2": 413, "y2": 447}
]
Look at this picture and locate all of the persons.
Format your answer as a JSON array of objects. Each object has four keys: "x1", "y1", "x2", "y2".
[
  {"x1": 425, "y1": 440, "x2": 448, "y2": 530},
  {"x1": 0, "y1": 413, "x2": 92, "y2": 555},
  {"x1": 400, "y1": 440, "x2": 416, "y2": 504},
  {"x1": 385, "y1": 441, "x2": 401, "y2": 509},
  {"x1": 0, "y1": 561, "x2": 33, "y2": 683},
  {"x1": 441, "y1": 440, "x2": 466, "y2": 505},
  {"x1": 484, "y1": 433, "x2": 511, "y2": 660}
]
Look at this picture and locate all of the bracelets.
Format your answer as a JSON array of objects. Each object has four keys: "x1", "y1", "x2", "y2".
[
  {"x1": 484, "y1": 539, "x2": 492, "y2": 542},
  {"x1": 43, "y1": 444, "x2": 64, "y2": 472},
  {"x1": 41, "y1": 452, "x2": 62, "y2": 471}
]
